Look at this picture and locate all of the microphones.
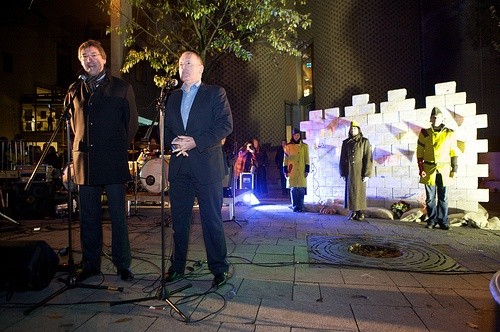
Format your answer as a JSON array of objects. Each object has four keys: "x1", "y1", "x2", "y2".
[
  {"x1": 163, "y1": 79, "x2": 178, "y2": 86},
  {"x1": 67, "y1": 75, "x2": 85, "y2": 92}
]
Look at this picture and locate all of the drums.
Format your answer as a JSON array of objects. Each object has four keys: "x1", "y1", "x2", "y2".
[
  {"x1": 139, "y1": 158, "x2": 170, "y2": 193},
  {"x1": 62, "y1": 162, "x2": 79, "y2": 193}
]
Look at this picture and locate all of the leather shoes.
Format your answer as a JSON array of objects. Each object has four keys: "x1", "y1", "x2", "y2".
[
  {"x1": 211, "y1": 271, "x2": 229, "y2": 287},
  {"x1": 72, "y1": 264, "x2": 101, "y2": 280},
  {"x1": 113, "y1": 265, "x2": 134, "y2": 281},
  {"x1": 161, "y1": 269, "x2": 185, "y2": 281}
]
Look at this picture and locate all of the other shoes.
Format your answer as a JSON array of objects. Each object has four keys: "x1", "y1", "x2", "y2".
[
  {"x1": 358, "y1": 213, "x2": 365, "y2": 221},
  {"x1": 347, "y1": 212, "x2": 356, "y2": 220},
  {"x1": 425, "y1": 223, "x2": 435, "y2": 229},
  {"x1": 440, "y1": 223, "x2": 449, "y2": 230},
  {"x1": 292, "y1": 206, "x2": 303, "y2": 212}
]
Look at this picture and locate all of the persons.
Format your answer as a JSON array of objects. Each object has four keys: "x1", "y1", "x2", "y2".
[
  {"x1": 63, "y1": 40, "x2": 140, "y2": 286},
  {"x1": 416, "y1": 107, "x2": 458, "y2": 230},
  {"x1": 339, "y1": 121, "x2": 373, "y2": 221},
  {"x1": 233, "y1": 141, "x2": 259, "y2": 189},
  {"x1": 163, "y1": 51, "x2": 234, "y2": 289},
  {"x1": 252, "y1": 137, "x2": 269, "y2": 199},
  {"x1": 282, "y1": 128, "x2": 310, "y2": 213},
  {"x1": 275, "y1": 140, "x2": 290, "y2": 198}
]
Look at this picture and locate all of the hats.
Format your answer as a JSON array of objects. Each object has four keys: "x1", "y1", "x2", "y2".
[
  {"x1": 350, "y1": 121, "x2": 360, "y2": 128},
  {"x1": 431, "y1": 107, "x2": 442, "y2": 117},
  {"x1": 291, "y1": 127, "x2": 299, "y2": 135}
]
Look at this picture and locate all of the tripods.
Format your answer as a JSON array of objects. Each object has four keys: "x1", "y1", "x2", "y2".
[
  {"x1": 23, "y1": 90, "x2": 125, "y2": 316},
  {"x1": 109, "y1": 91, "x2": 193, "y2": 324}
]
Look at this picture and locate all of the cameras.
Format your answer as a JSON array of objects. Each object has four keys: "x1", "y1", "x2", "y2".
[{"x1": 249, "y1": 146, "x2": 255, "y2": 150}]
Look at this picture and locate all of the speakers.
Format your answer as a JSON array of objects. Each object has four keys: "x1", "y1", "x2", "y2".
[{"x1": 0, "y1": 240, "x2": 60, "y2": 293}]
